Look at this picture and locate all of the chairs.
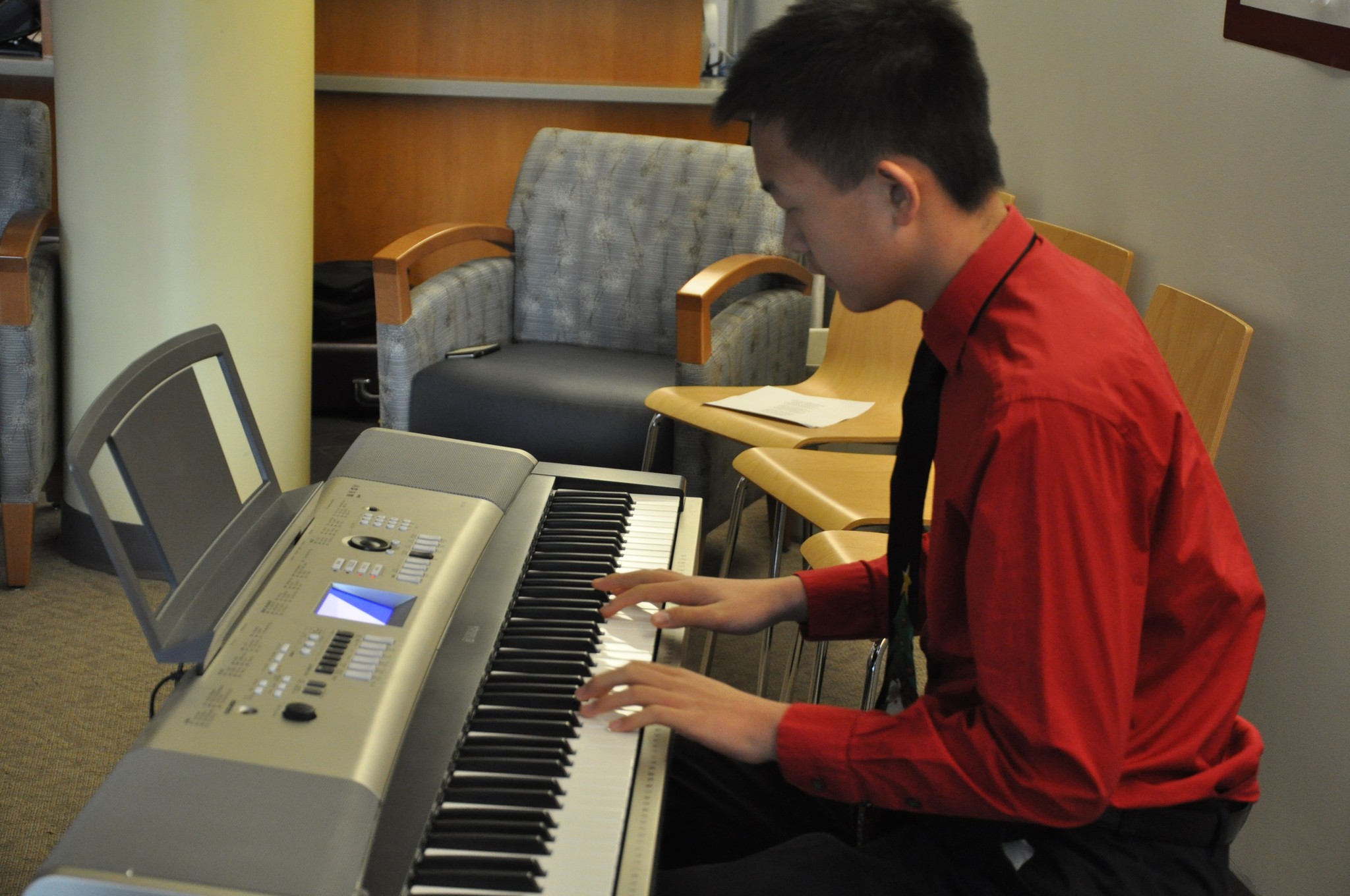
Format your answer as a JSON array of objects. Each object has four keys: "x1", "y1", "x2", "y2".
[
  {"x1": 0, "y1": 92, "x2": 71, "y2": 592},
  {"x1": 376, "y1": 129, "x2": 1252, "y2": 704}
]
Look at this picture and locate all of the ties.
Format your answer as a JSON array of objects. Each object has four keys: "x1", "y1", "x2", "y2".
[{"x1": 856, "y1": 336, "x2": 945, "y2": 846}]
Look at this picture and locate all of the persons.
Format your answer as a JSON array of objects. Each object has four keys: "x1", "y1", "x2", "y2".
[{"x1": 573, "y1": 0, "x2": 1269, "y2": 896}]
